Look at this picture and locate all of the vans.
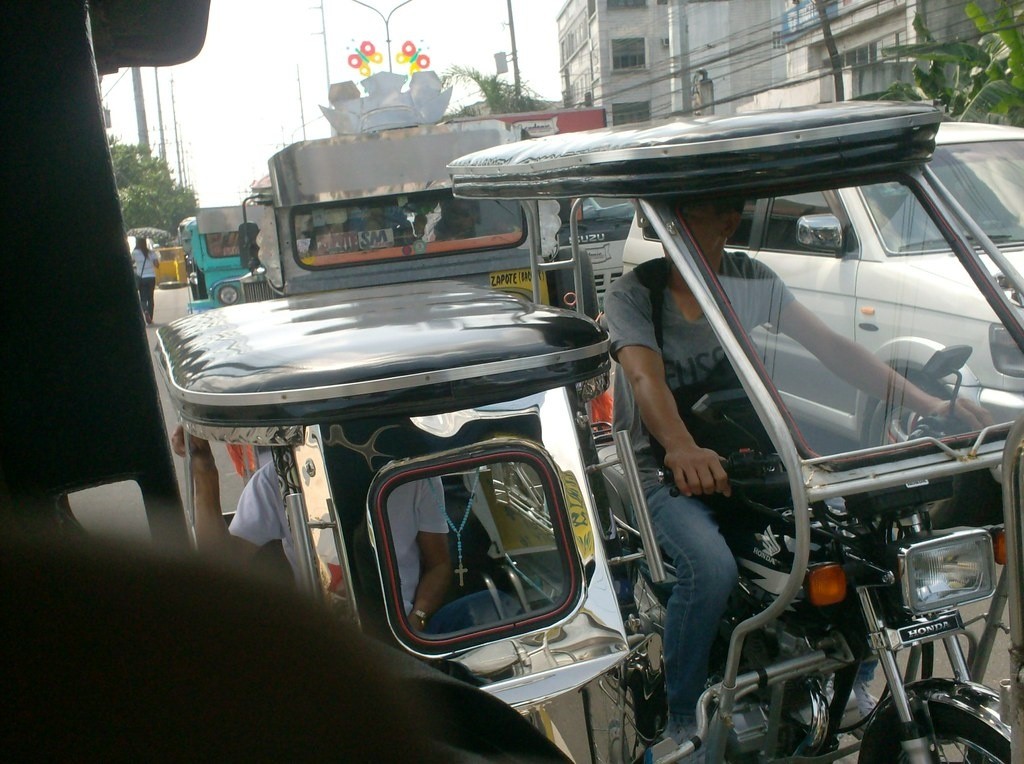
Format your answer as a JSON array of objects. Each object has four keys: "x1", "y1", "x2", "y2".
[
  {"x1": 177, "y1": 215, "x2": 262, "y2": 310},
  {"x1": 240, "y1": 42, "x2": 563, "y2": 312},
  {"x1": 622, "y1": 119, "x2": 1024, "y2": 464}
]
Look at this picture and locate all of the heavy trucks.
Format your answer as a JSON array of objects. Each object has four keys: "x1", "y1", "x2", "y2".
[{"x1": 442, "y1": 107, "x2": 637, "y2": 314}]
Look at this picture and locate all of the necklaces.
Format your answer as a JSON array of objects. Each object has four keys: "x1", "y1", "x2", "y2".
[{"x1": 428, "y1": 475, "x2": 480, "y2": 587}]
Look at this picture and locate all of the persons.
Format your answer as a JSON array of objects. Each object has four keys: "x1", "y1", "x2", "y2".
[
  {"x1": 131, "y1": 236, "x2": 159, "y2": 324},
  {"x1": 172, "y1": 423, "x2": 508, "y2": 670},
  {"x1": 606, "y1": 184, "x2": 995, "y2": 753}
]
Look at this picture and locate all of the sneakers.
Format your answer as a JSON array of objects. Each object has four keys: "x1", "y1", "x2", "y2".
[{"x1": 145, "y1": 311, "x2": 151, "y2": 324}]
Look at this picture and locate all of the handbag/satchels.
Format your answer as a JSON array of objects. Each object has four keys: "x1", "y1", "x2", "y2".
[{"x1": 133, "y1": 271, "x2": 141, "y2": 291}]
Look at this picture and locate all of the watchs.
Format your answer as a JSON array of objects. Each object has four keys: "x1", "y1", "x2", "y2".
[{"x1": 412, "y1": 609, "x2": 428, "y2": 629}]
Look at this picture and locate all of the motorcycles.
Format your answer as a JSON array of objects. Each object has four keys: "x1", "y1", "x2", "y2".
[{"x1": 148, "y1": 96, "x2": 1023, "y2": 762}]
